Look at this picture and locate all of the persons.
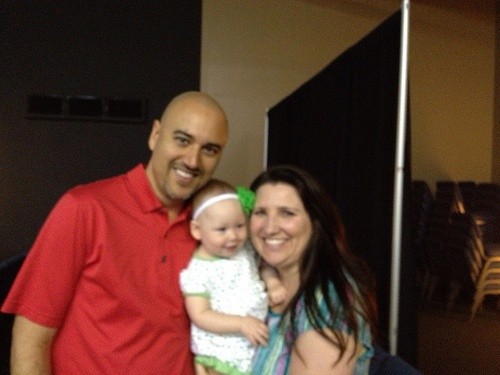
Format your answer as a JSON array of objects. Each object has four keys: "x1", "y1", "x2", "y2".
[{"x1": 0, "y1": 90, "x2": 379, "y2": 375}]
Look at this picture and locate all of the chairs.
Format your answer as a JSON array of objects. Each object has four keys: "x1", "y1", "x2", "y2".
[{"x1": 412, "y1": 180, "x2": 500, "y2": 322}]
